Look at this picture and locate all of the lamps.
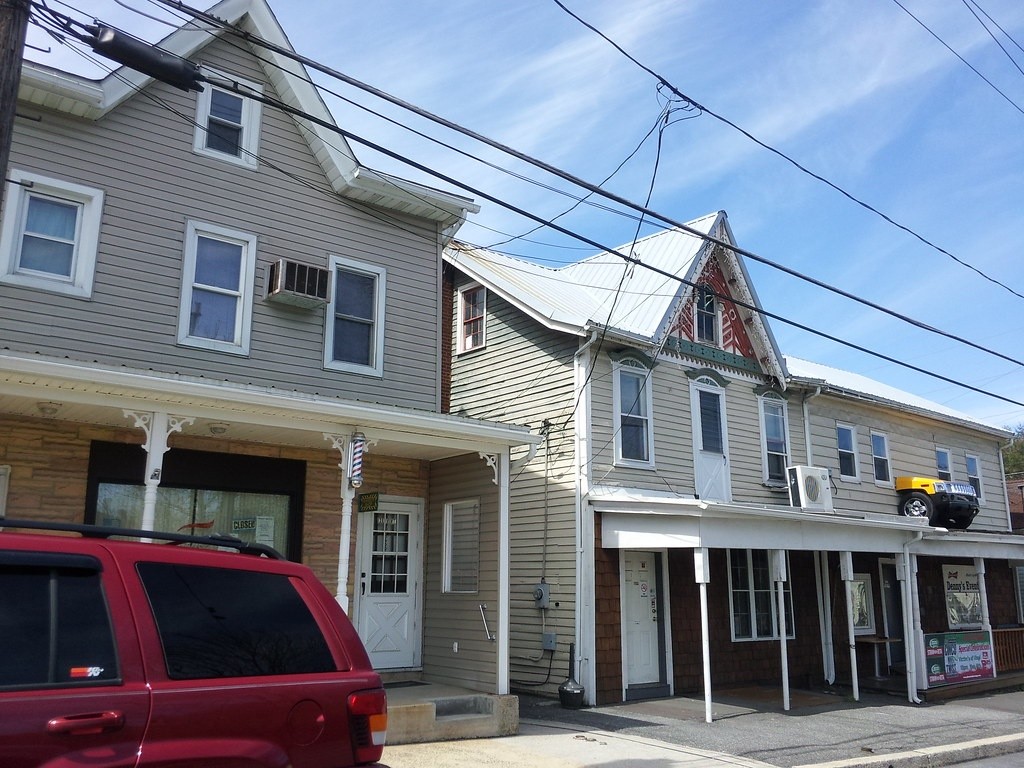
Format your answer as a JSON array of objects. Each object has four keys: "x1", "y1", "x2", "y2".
[
  {"x1": 37, "y1": 402, "x2": 63, "y2": 415},
  {"x1": 675, "y1": 340, "x2": 681, "y2": 352},
  {"x1": 209, "y1": 424, "x2": 231, "y2": 436},
  {"x1": 347, "y1": 431, "x2": 365, "y2": 489}
]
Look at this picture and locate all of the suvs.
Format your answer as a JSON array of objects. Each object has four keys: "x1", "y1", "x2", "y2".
[
  {"x1": 894, "y1": 475, "x2": 980, "y2": 530},
  {"x1": 0, "y1": 515, "x2": 388, "y2": 767}
]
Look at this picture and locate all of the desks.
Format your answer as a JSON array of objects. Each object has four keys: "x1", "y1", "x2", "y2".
[{"x1": 854, "y1": 636, "x2": 902, "y2": 681}]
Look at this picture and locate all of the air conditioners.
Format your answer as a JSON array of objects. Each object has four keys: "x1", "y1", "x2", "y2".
[
  {"x1": 785, "y1": 464, "x2": 834, "y2": 514},
  {"x1": 265, "y1": 259, "x2": 332, "y2": 309}
]
